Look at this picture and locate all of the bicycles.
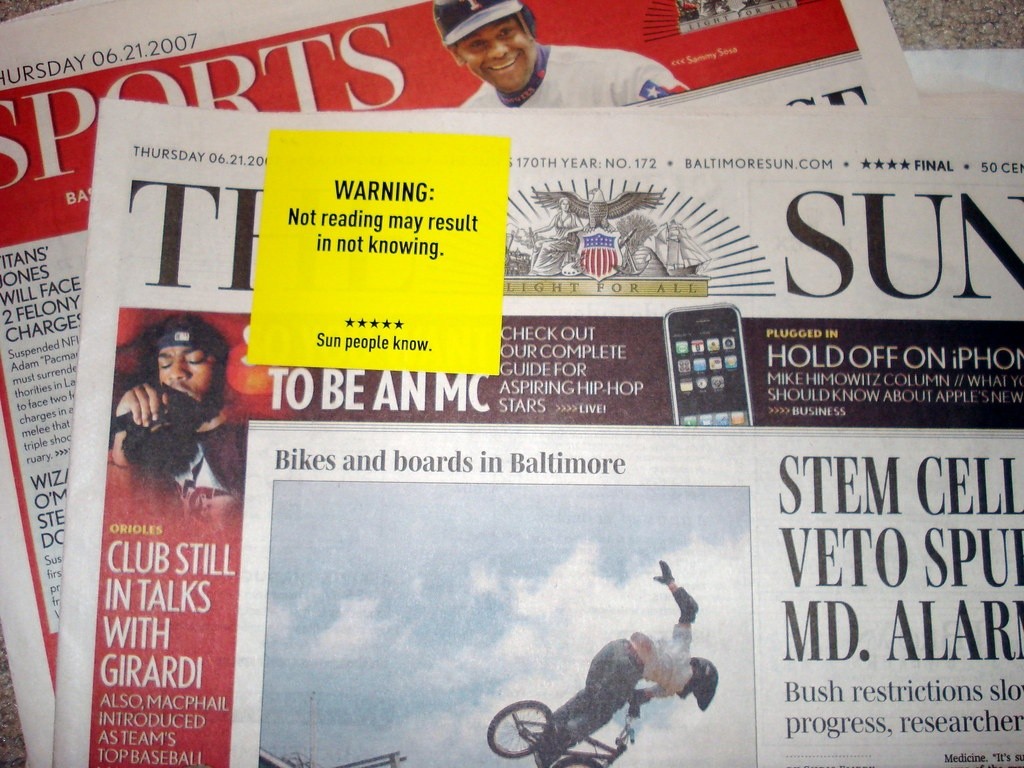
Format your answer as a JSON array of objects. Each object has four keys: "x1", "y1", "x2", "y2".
[{"x1": 487, "y1": 700, "x2": 635, "y2": 768}]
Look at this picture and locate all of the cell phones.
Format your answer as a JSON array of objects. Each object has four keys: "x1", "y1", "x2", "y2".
[{"x1": 664, "y1": 303, "x2": 753, "y2": 427}]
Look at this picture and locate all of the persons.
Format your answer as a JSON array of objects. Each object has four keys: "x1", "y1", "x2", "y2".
[
  {"x1": 112, "y1": 314, "x2": 301, "y2": 526},
  {"x1": 540, "y1": 560, "x2": 719, "y2": 764},
  {"x1": 432, "y1": 0, "x2": 690, "y2": 108}
]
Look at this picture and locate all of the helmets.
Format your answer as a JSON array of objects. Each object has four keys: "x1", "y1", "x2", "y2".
[
  {"x1": 679, "y1": 657, "x2": 717, "y2": 712},
  {"x1": 435, "y1": 0, "x2": 535, "y2": 45}
]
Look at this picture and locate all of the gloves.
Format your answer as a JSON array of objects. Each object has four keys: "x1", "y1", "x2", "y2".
[
  {"x1": 654, "y1": 560, "x2": 674, "y2": 586},
  {"x1": 628, "y1": 705, "x2": 641, "y2": 715}
]
